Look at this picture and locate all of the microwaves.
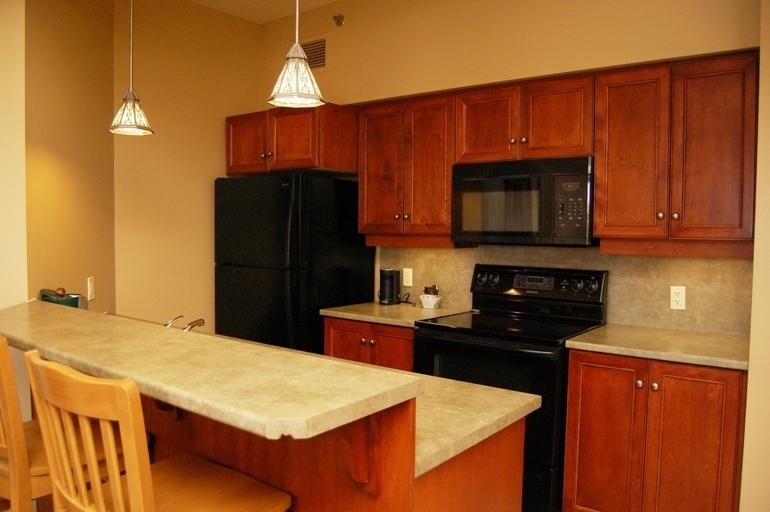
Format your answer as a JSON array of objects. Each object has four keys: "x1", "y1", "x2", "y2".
[{"x1": 448, "y1": 154, "x2": 593, "y2": 247}]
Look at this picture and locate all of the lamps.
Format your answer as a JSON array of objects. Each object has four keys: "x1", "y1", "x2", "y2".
[
  {"x1": 107, "y1": 0, "x2": 154, "y2": 136},
  {"x1": 266, "y1": 0, "x2": 327, "y2": 108}
]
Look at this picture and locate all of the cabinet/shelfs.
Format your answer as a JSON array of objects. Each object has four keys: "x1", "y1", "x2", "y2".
[
  {"x1": 324, "y1": 316, "x2": 414, "y2": 373},
  {"x1": 455, "y1": 69, "x2": 594, "y2": 165},
  {"x1": 561, "y1": 348, "x2": 747, "y2": 512},
  {"x1": 357, "y1": 88, "x2": 455, "y2": 248},
  {"x1": 225, "y1": 102, "x2": 359, "y2": 176},
  {"x1": 593, "y1": 47, "x2": 760, "y2": 259}
]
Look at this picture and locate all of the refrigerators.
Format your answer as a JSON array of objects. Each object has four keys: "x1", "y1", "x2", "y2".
[{"x1": 211, "y1": 175, "x2": 375, "y2": 355}]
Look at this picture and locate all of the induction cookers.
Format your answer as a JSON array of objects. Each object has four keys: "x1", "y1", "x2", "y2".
[{"x1": 411, "y1": 309, "x2": 611, "y2": 346}]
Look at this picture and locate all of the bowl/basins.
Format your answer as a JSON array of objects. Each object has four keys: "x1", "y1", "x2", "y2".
[{"x1": 419, "y1": 294, "x2": 443, "y2": 309}]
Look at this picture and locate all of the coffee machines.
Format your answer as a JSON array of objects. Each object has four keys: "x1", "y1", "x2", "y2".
[{"x1": 378, "y1": 266, "x2": 402, "y2": 306}]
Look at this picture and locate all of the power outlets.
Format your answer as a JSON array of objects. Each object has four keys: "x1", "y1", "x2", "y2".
[{"x1": 670, "y1": 285, "x2": 685, "y2": 310}]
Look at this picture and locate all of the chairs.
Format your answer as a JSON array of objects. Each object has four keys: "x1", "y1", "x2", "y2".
[
  {"x1": 24, "y1": 348, "x2": 292, "y2": 512},
  {"x1": 0, "y1": 334, "x2": 151, "y2": 512}
]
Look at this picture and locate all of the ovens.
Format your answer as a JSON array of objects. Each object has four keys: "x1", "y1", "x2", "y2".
[{"x1": 410, "y1": 335, "x2": 563, "y2": 512}]
